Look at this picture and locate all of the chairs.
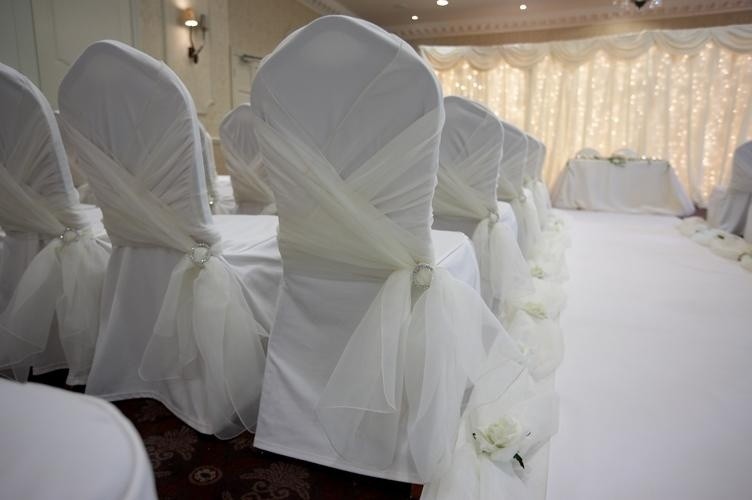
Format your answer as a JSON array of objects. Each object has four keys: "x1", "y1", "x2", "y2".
[{"x1": 707, "y1": 139, "x2": 752, "y2": 230}]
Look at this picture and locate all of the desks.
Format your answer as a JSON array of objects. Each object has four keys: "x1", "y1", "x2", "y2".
[{"x1": 563, "y1": 159, "x2": 672, "y2": 213}]
